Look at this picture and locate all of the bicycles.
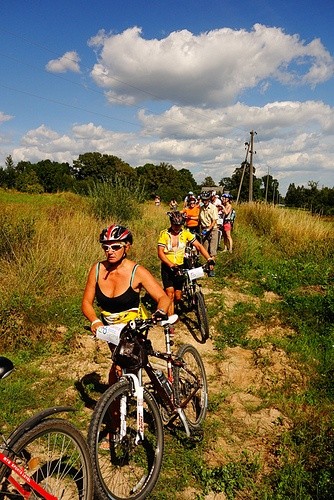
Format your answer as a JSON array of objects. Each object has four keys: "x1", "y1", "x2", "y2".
[
  {"x1": 171, "y1": 249, "x2": 214, "y2": 340},
  {"x1": 0, "y1": 356, "x2": 93, "y2": 500},
  {"x1": 84, "y1": 313, "x2": 210, "y2": 500}
]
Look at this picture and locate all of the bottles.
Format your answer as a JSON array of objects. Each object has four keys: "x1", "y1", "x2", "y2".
[{"x1": 155, "y1": 369, "x2": 174, "y2": 395}]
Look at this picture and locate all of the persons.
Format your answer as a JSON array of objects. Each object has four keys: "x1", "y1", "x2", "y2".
[
  {"x1": 81, "y1": 224, "x2": 171, "y2": 451},
  {"x1": 180, "y1": 191, "x2": 202, "y2": 243},
  {"x1": 210, "y1": 190, "x2": 233, "y2": 253},
  {"x1": 159, "y1": 210, "x2": 215, "y2": 338},
  {"x1": 199, "y1": 191, "x2": 219, "y2": 262},
  {"x1": 155, "y1": 197, "x2": 160, "y2": 205},
  {"x1": 169, "y1": 199, "x2": 179, "y2": 210}
]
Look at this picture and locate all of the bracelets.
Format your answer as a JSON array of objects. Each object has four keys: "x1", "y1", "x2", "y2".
[{"x1": 91, "y1": 319, "x2": 100, "y2": 326}]
